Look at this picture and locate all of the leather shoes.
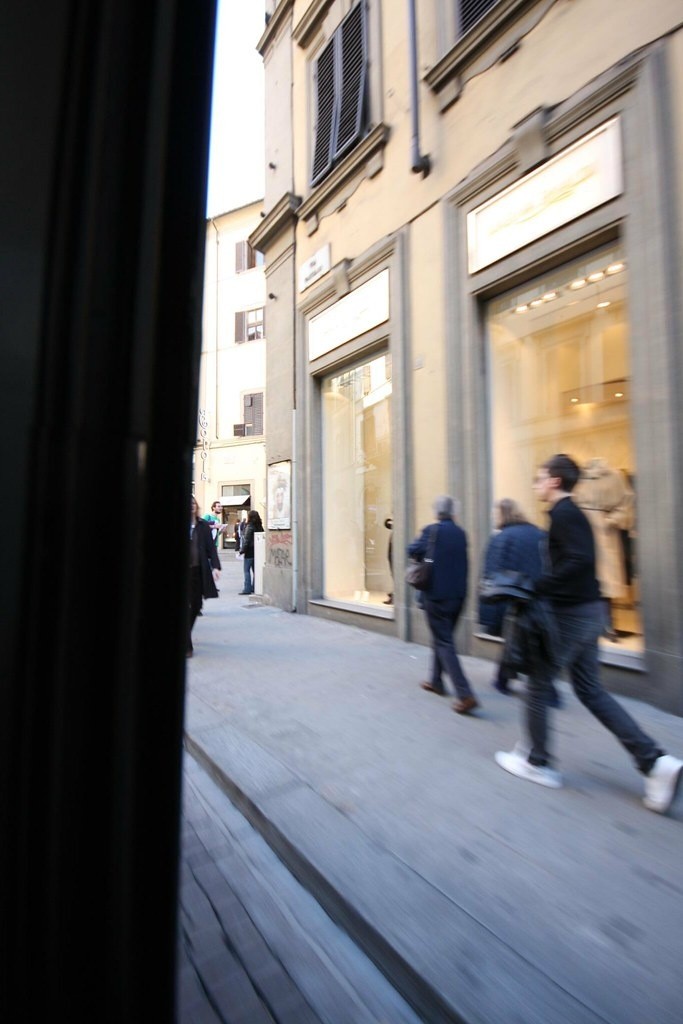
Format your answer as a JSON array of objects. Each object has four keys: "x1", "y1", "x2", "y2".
[
  {"x1": 420, "y1": 681, "x2": 441, "y2": 695},
  {"x1": 450, "y1": 697, "x2": 477, "y2": 712}
]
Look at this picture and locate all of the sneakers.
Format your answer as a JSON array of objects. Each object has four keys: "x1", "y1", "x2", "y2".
[
  {"x1": 494, "y1": 751, "x2": 562, "y2": 789},
  {"x1": 642, "y1": 754, "x2": 682, "y2": 813}
]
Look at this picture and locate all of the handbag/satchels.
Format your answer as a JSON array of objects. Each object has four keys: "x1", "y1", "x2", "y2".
[{"x1": 405, "y1": 524, "x2": 439, "y2": 589}]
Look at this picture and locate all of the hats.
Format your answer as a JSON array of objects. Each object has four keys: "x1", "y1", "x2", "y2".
[{"x1": 430, "y1": 496, "x2": 456, "y2": 516}]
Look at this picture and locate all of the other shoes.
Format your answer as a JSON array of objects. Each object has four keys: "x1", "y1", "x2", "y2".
[
  {"x1": 185, "y1": 649, "x2": 192, "y2": 658},
  {"x1": 491, "y1": 680, "x2": 508, "y2": 695},
  {"x1": 383, "y1": 600, "x2": 392, "y2": 605},
  {"x1": 387, "y1": 593, "x2": 393, "y2": 597},
  {"x1": 238, "y1": 592, "x2": 250, "y2": 595},
  {"x1": 544, "y1": 695, "x2": 558, "y2": 707}
]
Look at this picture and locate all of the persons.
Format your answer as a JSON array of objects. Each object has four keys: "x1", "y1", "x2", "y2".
[
  {"x1": 576, "y1": 458, "x2": 633, "y2": 641},
  {"x1": 382, "y1": 517, "x2": 394, "y2": 605},
  {"x1": 495, "y1": 453, "x2": 683, "y2": 815},
  {"x1": 407, "y1": 493, "x2": 479, "y2": 716},
  {"x1": 271, "y1": 480, "x2": 290, "y2": 520},
  {"x1": 185, "y1": 496, "x2": 264, "y2": 655},
  {"x1": 482, "y1": 499, "x2": 557, "y2": 708}
]
